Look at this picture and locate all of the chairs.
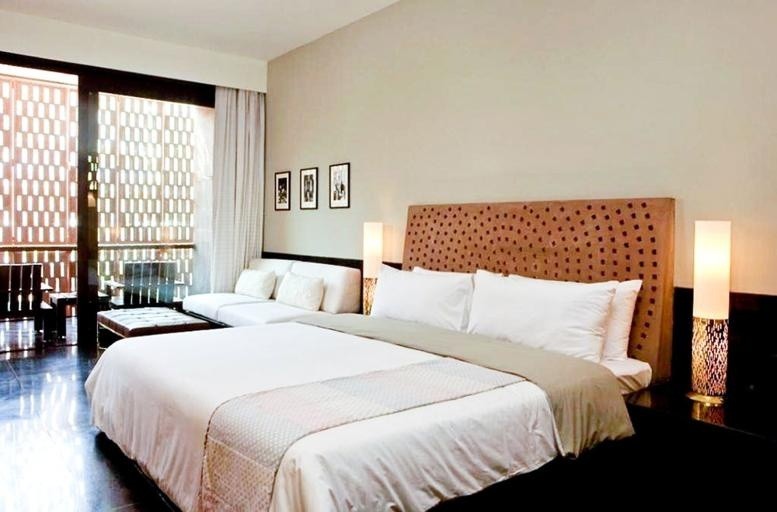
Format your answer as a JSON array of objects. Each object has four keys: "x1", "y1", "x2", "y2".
[
  {"x1": 104, "y1": 263, "x2": 185, "y2": 309},
  {"x1": 0, "y1": 264, "x2": 55, "y2": 344}
]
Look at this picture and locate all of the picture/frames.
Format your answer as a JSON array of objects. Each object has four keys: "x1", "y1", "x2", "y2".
[
  {"x1": 274, "y1": 171, "x2": 291, "y2": 212},
  {"x1": 328, "y1": 163, "x2": 350, "y2": 209},
  {"x1": 300, "y1": 167, "x2": 318, "y2": 210}
]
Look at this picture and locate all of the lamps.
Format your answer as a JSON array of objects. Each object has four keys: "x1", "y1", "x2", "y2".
[
  {"x1": 362, "y1": 223, "x2": 385, "y2": 315},
  {"x1": 686, "y1": 219, "x2": 732, "y2": 409}
]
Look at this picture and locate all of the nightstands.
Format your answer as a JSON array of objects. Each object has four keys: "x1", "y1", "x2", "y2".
[{"x1": 622, "y1": 378, "x2": 776, "y2": 512}]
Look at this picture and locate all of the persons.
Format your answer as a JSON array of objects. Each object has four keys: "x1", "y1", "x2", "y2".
[
  {"x1": 303, "y1": 174, "x2": 315, "y2": 203},
  {"x1": 334, "y1": 179, "x2": 346, "y2": 200},
  {"x1": 278, "y1": 185, "x2": 286, "y2": 203}
]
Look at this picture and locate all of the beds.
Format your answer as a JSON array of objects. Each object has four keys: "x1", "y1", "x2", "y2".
[{"x1": 84, "y1": 197, "x2": 677, "y2": 512}]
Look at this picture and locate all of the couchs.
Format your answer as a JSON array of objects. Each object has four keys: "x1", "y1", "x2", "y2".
[{"x1": 183, "y1": 259, "x2": 361, "y2": 327}]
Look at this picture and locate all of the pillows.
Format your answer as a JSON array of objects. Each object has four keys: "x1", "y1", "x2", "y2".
[
  {"x1": 276, "y1": 273, "x2": 325, "y2": 311},
  {"x1": 234, "y1": 270, "x2": 276, "y2": 300},
  {"x1": 376, "y1": 265, "x2": 644, "y2": 366}
]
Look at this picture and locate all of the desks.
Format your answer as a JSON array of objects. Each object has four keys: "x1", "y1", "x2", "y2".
[{"x1": 49, "y1": 292, "x2": 108, "y2": 342}]
